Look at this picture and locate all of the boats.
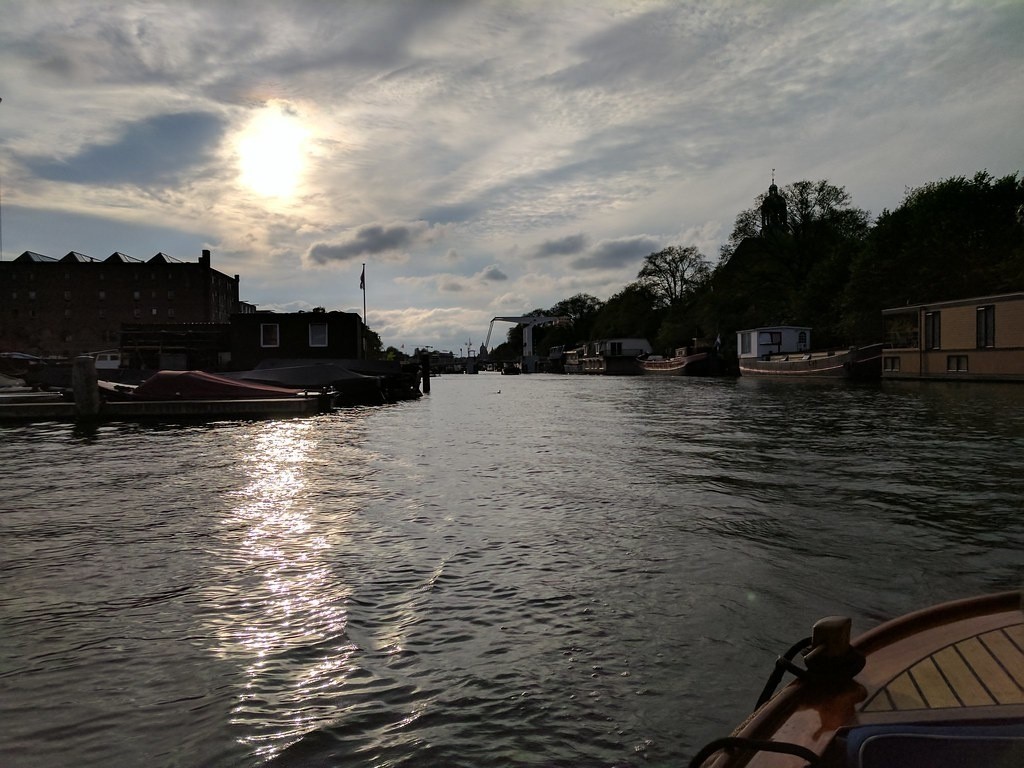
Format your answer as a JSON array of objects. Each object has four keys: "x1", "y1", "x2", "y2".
[
  {"x1": 313, "y1": 347, "x2": 423, "y2": 404},
  {"x1": 735, "y1": 325, "x2": 884, "y2": 379},
  {"x1": 635, "y1": 346, "x2": 709, "y2": 377},
  {"x1": 97, "y1": 370, "x2": 341, "y2": 413}
]
